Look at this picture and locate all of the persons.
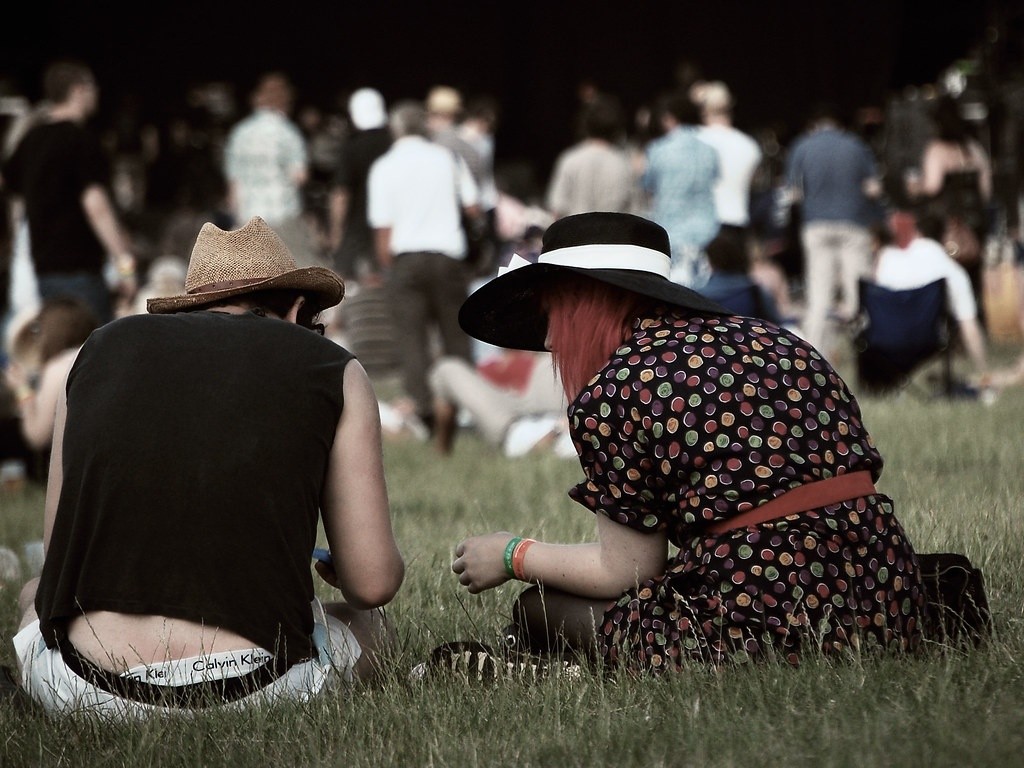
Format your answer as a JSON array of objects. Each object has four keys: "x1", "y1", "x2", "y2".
[
  {"x1": 12, "y1": 216, "x2": 405, "y2": 724},
  {"x1": 452, "y1": 212, "x2": 948, "y2": 682},
  {"x1": 0, "y1": 61, "x2": 1024, "y2": 491}
]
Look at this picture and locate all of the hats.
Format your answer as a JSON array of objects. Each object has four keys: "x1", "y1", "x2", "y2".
[
  {"x1": 457, "y1": 212, "x2": 741, "y2": 352},
  {"x1": 349, "y1": 88, "x2": 387, "y2": 130},
  {"x1": 146, "y1": 216, "x2": 345, "y2": 314}
]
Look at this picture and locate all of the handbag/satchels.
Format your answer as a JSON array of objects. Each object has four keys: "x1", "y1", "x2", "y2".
[{"x1": 915, "y1": 553, "x2": 1000, "y2": 646}]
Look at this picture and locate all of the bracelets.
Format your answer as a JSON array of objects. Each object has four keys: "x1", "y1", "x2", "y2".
[{"x1": 503, "y1": 536, "x2": 537, "y2": 583}]
